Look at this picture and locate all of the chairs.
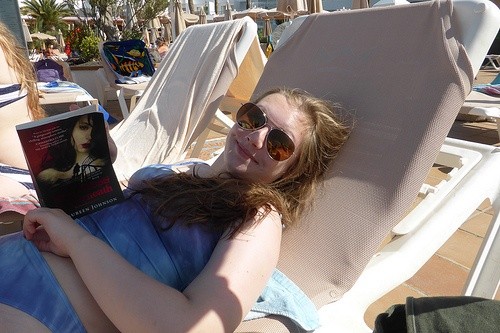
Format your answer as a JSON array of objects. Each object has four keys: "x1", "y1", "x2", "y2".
[{"x1": 0, "y1": 0, "x2": 500, "y2": 333}]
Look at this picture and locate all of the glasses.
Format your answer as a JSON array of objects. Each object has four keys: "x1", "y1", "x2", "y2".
[{"x1": 236, "y1": 103, "x2": 300, "y2": 163}]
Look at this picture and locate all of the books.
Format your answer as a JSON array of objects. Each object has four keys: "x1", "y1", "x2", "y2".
[{"x1": 15, "y1": 105, "x2": 126, "y2": 221}]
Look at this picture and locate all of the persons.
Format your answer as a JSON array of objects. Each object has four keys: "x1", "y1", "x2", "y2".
[
  {"x1": 0, "y1": 22, "x2": 47, "y2": 197},
  {"x1": 0, "y1": 86, "x2": 352, "y2": 333},
  {"x1": 34, "y1": 43, "x2": 68, "y2": 82},
  {"x1": 141, "y1": 34, "x2": 169, "y2": 60},
  {"x1": 37, "y1": 115, "x2": 108, "y2": 181}
]
